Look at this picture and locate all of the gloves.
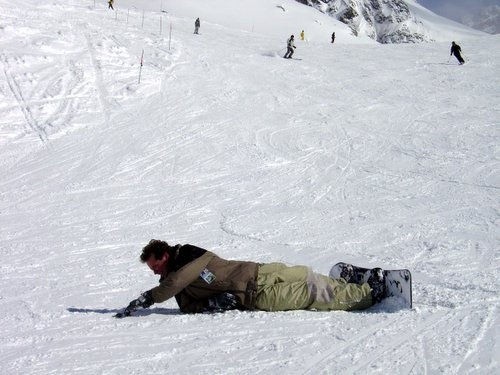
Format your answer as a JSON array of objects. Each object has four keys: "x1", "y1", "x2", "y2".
[
  {"x1": 124, "y1": 291, "x2": 152, "y2": 316},
  {"x1": 205, "y1": 292, "x2": 239, "y2": 311}
]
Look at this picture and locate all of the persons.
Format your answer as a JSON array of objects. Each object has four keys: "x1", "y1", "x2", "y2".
[
  {"x1": 193, "y1": 18, "x2": 203, "y2": 34},
  {"x1": 448, "y1": 41, "x2": 464, "y2": 65},
  {"x1": 299, "y1": 28, "x2": 306, "y2": 43},
  {"x1": 329, "y1": 31, "x2": 338, "y2": 45},
  {"x1": 106, "y1": 0, "x2": 115, "y2": 10},
  {"x1": 113, "y1": 239, "x2": 386, "y2": 318},
  {"x1": 283, "y1": 33, "x2": 297, "y2": 59}
]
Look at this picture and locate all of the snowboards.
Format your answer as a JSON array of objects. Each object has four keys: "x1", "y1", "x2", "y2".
[{"x1": 329, "y1": 262, "x2": 412, "y2": 309}]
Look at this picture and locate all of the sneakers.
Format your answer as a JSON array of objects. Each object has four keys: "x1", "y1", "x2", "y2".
[{"x1": 367, "y1": 268, "x2": 386, "y2": 305}]
